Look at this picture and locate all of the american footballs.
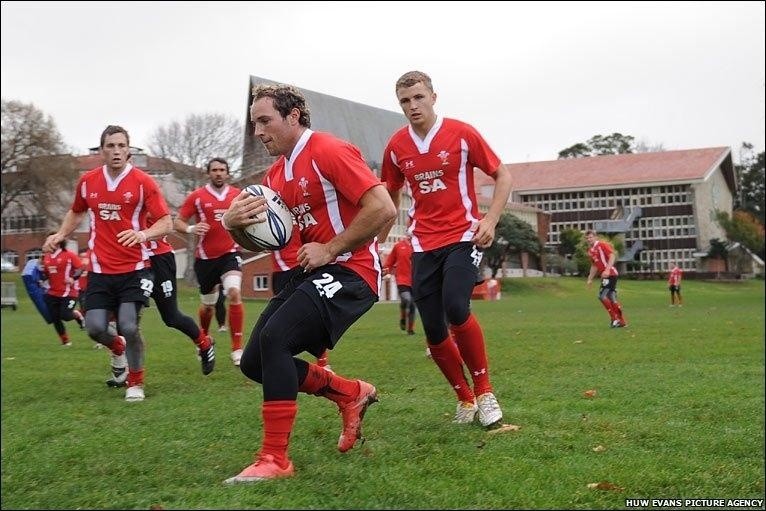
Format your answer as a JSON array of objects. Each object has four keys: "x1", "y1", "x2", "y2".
[{"x1": 243, "y1": 185, "x2": 293, "y2": 249}]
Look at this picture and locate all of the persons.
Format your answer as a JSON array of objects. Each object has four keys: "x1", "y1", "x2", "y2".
[
  {"x1": 586, "y1": 231, "x2": 626, "y2": 328},
  {"x1": 37, "y1": 71, "x2": 513, "y2": 485},
  {"x1": 668, "y1": 260, "x2": 684, "y2": 307}
]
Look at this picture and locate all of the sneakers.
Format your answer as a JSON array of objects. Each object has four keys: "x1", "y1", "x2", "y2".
[
  {"x1": 222, "y1": 449, "x2": 295, "y2": 488},
  {"x1": 609, "y1": 318, "x2": 627, "y2": 328},
  {"x1": 195, "y1": 335, "x2": 216, "y2": 375},
  {"x1": 322, "y1": 364, "x2": 379, "y2": 452},
  {"x1": 230, "y1": 348, "x2": 244, "y2": 367},
  {"x1": 218, "y1": 323, "x2": 226, "y2": 332},
  {"x1": 476, "y1": 392, "x2": 503, "y2": 428},
  {"x1": 60, "y1": 309, "x2": 145, "y2": 402},
  {"x1": 399, "y1": 318, "x2": 415, "y2": 335},
  {"x1": 451, "y1": 392, "x2": 478, "y2": 425}
]
